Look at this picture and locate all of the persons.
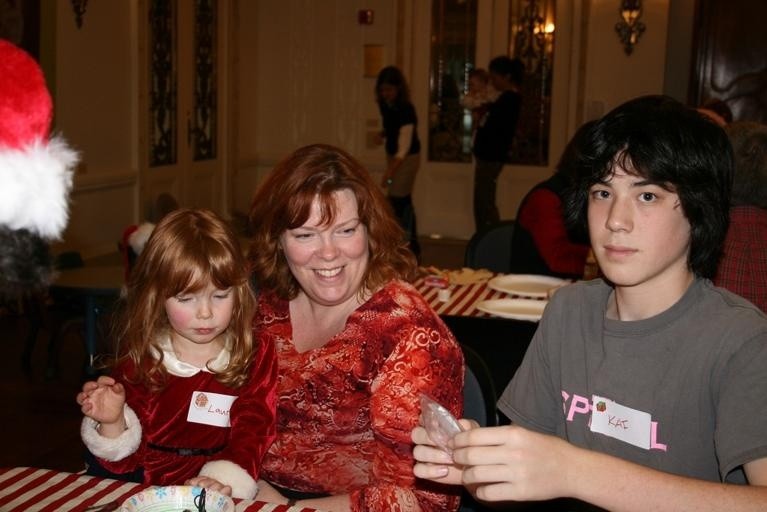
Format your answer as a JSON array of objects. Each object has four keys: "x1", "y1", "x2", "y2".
[
  {"x1": 469, "y1": 55, "x2": 524, "y2": 232},
  {"x1": 242, "y1": 140, "x2": 469, "y2": 512},
  {"x1": 503, "y1": 116, "x2": 604, "y2": 283},
  {"x1": 410, "y1": 91, "x2": 765, "y2": 512},
  {"x1": 459, "y1": 68, "x2": 500, "y2": 140},
  {"x1": 73, "y1": 207, "x2": 278, "y2": 505},
  {"x1": 365, "y1": 65, "x2": 422, "y2": 255}
]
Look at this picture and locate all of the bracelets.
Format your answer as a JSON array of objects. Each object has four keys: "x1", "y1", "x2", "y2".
[{"x1": 286, "y1": 498, "x2": 298, "y2": 506}]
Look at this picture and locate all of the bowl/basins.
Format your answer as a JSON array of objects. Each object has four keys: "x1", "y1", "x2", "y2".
[{"x1": 121, "y1": 484, "x2": 236, "y2": 512}]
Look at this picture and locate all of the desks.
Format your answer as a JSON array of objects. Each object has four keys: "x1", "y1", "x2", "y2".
[{"x1": 45, "y1": 266, "x2": 127, "y2": 381}]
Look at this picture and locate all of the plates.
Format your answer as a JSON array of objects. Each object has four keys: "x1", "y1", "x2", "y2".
[
  {"x1": 476, "y1": 298, "x2": 550, "y2": 320},
  {"x1": 488, "y1": 274, "x2": 569, "y2": 298}
]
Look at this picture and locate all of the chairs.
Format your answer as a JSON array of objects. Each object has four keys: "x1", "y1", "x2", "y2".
[
  {"x1": 462, "y1": 219, "x2": 518, "y2": 271},
  {"x1": 458, "y1": 344, "x2": 500, "y2": 431}
]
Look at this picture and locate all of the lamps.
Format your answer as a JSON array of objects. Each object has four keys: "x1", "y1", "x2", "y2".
[{"x1": 615, "y1": 1, "x2": 648, "y2": 56}]
